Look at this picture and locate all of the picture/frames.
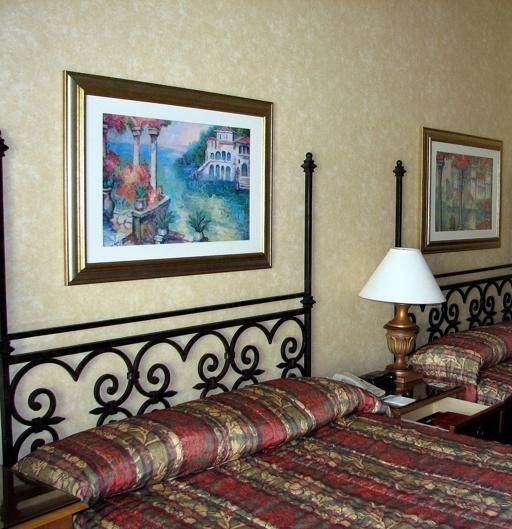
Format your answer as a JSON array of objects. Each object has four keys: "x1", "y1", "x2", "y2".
[
  {"x1": 59, "y1": 70, "x2": 274, "y2": 288},
  {"x1": 417, "y1": 124, "x2": 505, "y2": 256}
]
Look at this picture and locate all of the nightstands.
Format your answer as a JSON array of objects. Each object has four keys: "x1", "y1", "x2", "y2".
[{"x1": 351, "y1": 368, "x2": 507, "y2": 440}]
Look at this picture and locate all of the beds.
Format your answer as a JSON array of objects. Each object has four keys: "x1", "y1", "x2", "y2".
[
  {"x1": 2, "y1": 294, "x2": 511, "y2": 528},
  {"x1": 391, "y1": 260, "x2": 512, "y2": 444}
]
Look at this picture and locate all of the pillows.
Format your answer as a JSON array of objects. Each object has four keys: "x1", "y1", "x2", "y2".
[
  {"x1": 13, "y1": 369, "x2": 394, "y2": 503},
  {"x1": 405, "y1": 319, "x2": 512, "y2": 386}
]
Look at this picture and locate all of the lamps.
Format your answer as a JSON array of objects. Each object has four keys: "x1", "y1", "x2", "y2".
[{"x1": 354, "y1": 242, "x2": 448, "y2": 388}]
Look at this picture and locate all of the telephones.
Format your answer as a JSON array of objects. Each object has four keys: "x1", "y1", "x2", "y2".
[{"x1": 331, "y1": 371, "x2": 386, "y2": 396}]
